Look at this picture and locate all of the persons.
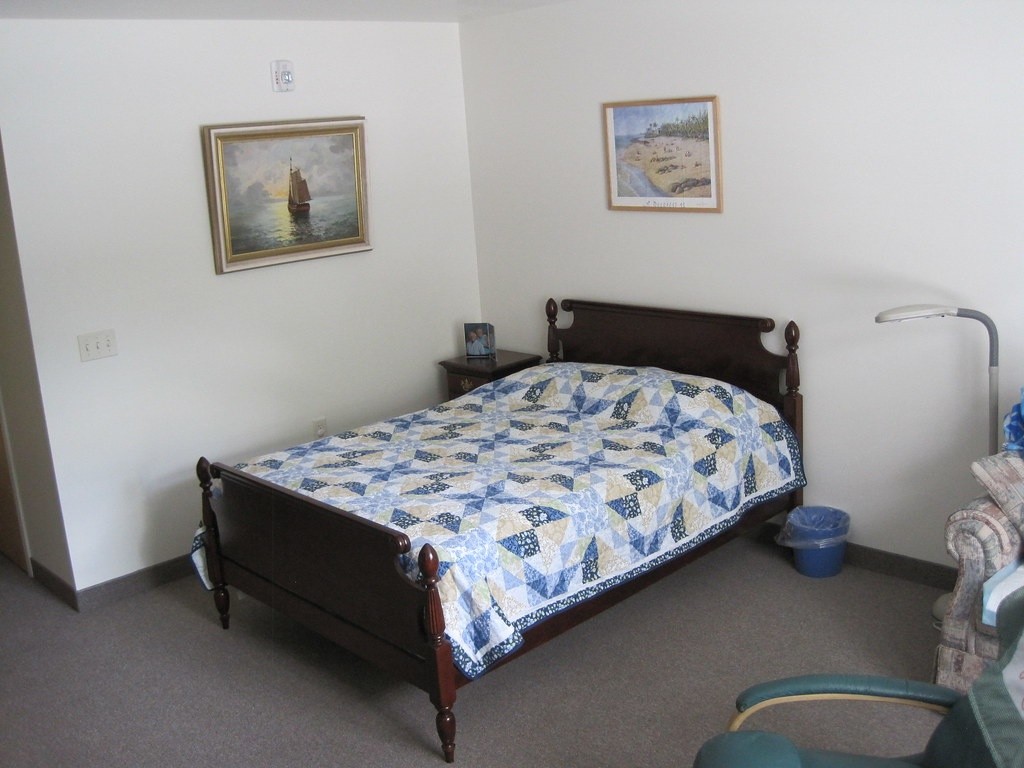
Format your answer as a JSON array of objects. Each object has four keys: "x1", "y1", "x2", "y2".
[{"x1": 466, "y1": 326, "x2": 489, "y2": 355}]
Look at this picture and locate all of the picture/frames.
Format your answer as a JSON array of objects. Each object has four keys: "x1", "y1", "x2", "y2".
[
  {"x1": 203, "y1": 116, "x2": 374, "y2": 275},
  {"x1": 464, "y1": 323, "x2": 488, "y2": 358},
  {"x1": 603, "y1": 95, "x2": 724, "y2": 214},
  {"x1": 487, "y1": 324, "x2": 498, "y2": 362}
]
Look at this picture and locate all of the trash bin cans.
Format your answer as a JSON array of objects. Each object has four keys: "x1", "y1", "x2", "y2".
[{"x1": 785, "y1": 504, "x2": 850, "y2": 577}]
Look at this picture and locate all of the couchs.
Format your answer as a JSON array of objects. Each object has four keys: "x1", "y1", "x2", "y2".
[{"x1": 935, "y1": 388, "x2": 1024, "y2": 697}]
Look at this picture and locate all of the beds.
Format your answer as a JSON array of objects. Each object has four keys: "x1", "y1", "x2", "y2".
[{"x1": 191, "y1": 297, "x2": 807, "y2": 764}]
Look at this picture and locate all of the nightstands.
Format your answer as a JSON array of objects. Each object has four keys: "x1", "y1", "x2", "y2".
[{"x1": 438, "y1": 350, "x2": 543, "y2": 402}]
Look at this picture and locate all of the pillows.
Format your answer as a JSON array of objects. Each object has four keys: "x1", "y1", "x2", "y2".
[{"x1": 967, "y1": 449, "x2": 1024, "y2": 538}]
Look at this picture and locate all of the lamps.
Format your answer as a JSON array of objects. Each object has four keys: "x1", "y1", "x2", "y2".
[{"x1": 875, "y1": 304, "x2": 999, "y2": 630}]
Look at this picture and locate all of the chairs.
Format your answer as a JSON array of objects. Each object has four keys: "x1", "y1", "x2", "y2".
[{"x1": 692, "y1": 585, "x2": 1024, "y2": 768}]
